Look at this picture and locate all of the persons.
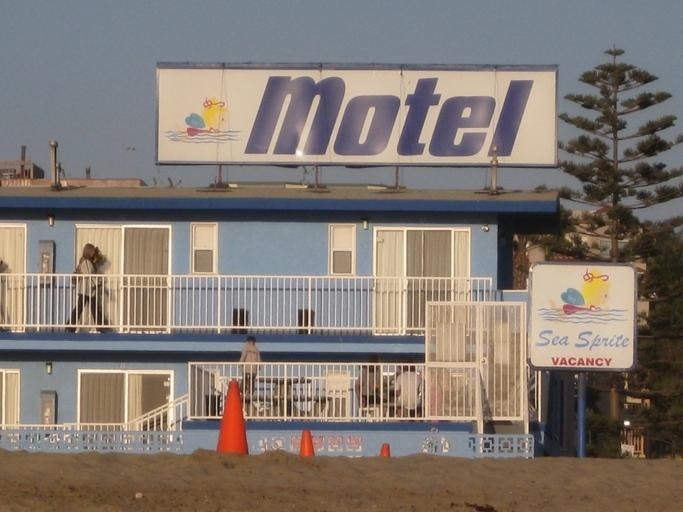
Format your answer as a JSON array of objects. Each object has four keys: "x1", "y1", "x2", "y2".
[
  {"x1": 389, "y1": 366, "x2": 404, "y2": 399},
  {"x1": 236, "y1": 336, "x2": 261, "y2": 394},
  {"x1": 359, "y1": 356, "x2": 390, "y2": 403},
  {"x1": 392, "y1": 362, "x2": 422, "y2": 417},
  {"x1": 354, "y1": 365, "x2": 374, "y2": 408},
  {"x1": 63, "y1": 243, "x2": 109, "y2": 333}
]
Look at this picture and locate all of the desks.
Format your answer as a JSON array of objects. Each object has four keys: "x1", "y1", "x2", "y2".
[{"x1": 231, "y1": 378, "x2": 313, "y2": 417}]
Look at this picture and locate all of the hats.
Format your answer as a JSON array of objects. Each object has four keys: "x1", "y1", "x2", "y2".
[{"x1": 246, "y1": 336, "x2": 256, "y2": 341}]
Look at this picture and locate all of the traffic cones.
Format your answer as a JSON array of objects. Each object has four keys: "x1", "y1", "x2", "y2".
[
  {"x1": 218, "y1": 381, "x2": 250, "y2": 454},
  {"x1": 381, "y1": 443, "x2": 390, "y2": 456},
  {"x1": 301, "y1": 429, "x2": 314, "y2": 457}
]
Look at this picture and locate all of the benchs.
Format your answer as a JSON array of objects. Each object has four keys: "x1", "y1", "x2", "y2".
[
  {"x1": 359, "y1": 398, "x2": 422, "y2": 418},
  {"x1": 206, "y1": 395, "x2": 332, "y2": 417}
]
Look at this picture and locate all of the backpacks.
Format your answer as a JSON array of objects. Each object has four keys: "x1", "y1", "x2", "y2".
[{"x1": 72, "y1": 264, "x2": 81, "y2": 284}]
